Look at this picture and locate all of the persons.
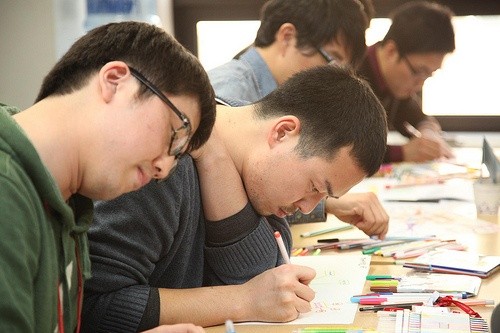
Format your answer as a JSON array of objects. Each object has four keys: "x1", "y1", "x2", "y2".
[
  {"x1": 81, "y1": 66, "x2": 389, "y2": 332},
  {"x1": 212, "y1": 0, "x2": 389, "y2": 242},
  {"x1": 0, "y1": 22, "x2": 217, "y2": 332},
  {"x1": 359, "y1": 0, "x2": 455, "y2": 164}
]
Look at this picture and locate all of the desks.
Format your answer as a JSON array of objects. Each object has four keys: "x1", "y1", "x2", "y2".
[{"x1": 203, "y1": 148, "x2": 500, "y2": 333}]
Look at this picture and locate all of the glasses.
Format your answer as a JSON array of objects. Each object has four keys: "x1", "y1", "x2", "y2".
[
  {"x1": 126, "y1": 66, "x2": 192, "y2": 184},
  {"x1": 403, "y1": 57, "x2": 433, "y2": 78},
  {"x1": 311, "y1": 42, "x2": 343, "y2": 68}
]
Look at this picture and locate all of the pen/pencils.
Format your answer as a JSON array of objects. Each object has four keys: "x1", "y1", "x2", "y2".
[
  {"x1": 293, "y1": 327, "x2": 364, "y2": 333},
  {"x1": 403, "y1": 122, "x2": 449, "y2": 159},
  {"x1": 317, "y1": 238, "x2": 468, "y2": 264},
  {"x1": 226, "y1": 319, "x2": 235, "y2": 333},
  {"x1": 351, "y1": 275, "x2": 466, "y2": 312},
  {"x1": 385, "y1": 180, "x2": 445, "y2": 189},
  {"x1": 274, "y1": 231, "x2": 291, "y2": 264},
  {"x1": 300, "y1": 225, "x2": 354, "y2": 237},
  {"x1": 451, "y1": 300, "x2": 494, "y2": 306},
  {"x1": 290, "y1": 247, "x2": 321, "y2": 256}
]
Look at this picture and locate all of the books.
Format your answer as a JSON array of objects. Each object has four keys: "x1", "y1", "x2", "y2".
[
  {"x1": 404, "y1": 246, "x2": 500, "y2": 279},
  {"x1": 397, "y1": 272, "x2": 482, "y2": 297},
  {"x1": 343, "y1": 148, "x2": 484, "y2": 241}
]
